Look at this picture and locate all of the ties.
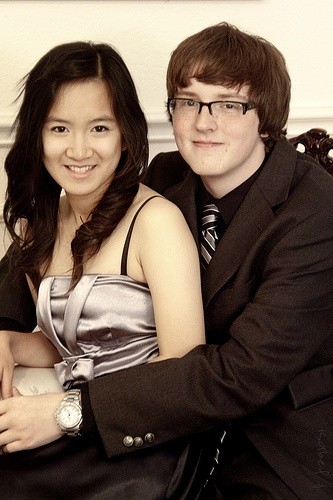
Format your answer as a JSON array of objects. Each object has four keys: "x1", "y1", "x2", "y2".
[{"x1": 200, "y1": 204, "x2": 220, "y2": 266}]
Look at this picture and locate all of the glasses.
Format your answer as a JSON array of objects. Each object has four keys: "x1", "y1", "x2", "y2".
[{"x1": 166, "y1": 97, "x2": 257, "y2": 119}]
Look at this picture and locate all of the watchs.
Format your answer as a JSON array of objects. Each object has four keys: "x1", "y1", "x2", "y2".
[{"x1": 56, "y1": 389, "x2": 83, "y2": 437}]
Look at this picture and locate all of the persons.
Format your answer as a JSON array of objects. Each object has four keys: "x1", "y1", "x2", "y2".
[
  {"x1": 0, "y1": 42, "x2": 208, "y2": 500},
  {"x1": 0, "y1": 20, "x2": 333, "y2": 500}
]
